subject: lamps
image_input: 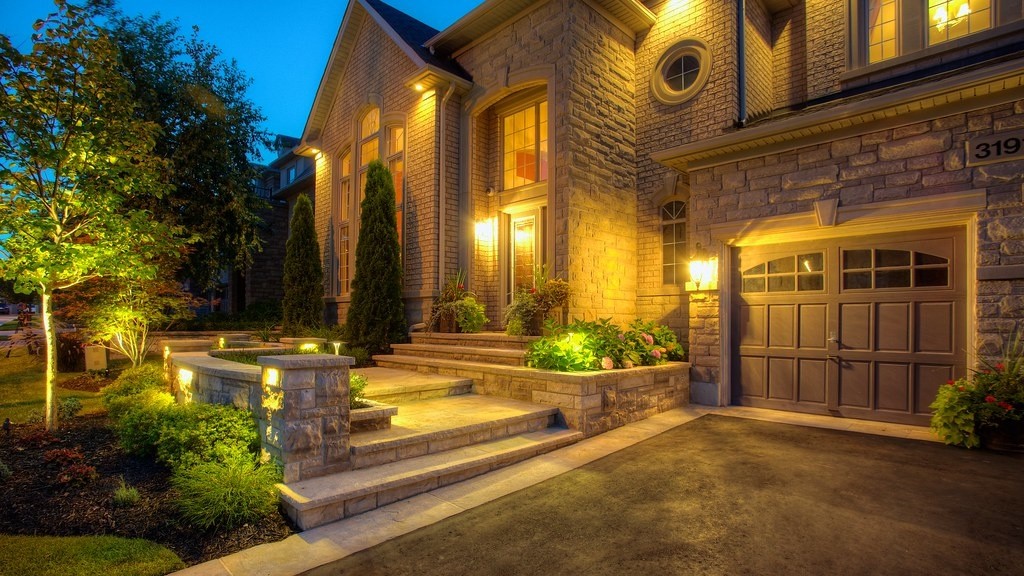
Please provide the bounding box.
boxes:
[934,0,971,32]
[689,242,708,292]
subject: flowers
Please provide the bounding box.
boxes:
[514,260,572,310]
[929,363,1024,449]
[525,316,687,370]
[431,267,479,307]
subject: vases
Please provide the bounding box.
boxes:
[530,310,564,335]
[440,307,457,333]
